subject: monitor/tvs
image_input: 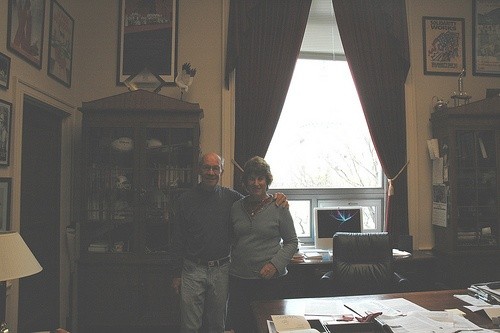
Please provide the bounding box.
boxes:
[313,208,364,249]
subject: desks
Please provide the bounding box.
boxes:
[246,285,500,333]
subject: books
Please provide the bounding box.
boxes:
[87,236,131,255]
[467,280,500,305]
[85,128,191,151]
[85,158,191,187]
[84,189,180,220]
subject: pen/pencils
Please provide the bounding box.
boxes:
[467,287,493,304]
[343,304,364,319]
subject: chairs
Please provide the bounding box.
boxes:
[319,229,408,291]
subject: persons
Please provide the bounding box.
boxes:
[229,155,300,333]
[167,150,286,332]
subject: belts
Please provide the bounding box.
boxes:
[185,255,231,267]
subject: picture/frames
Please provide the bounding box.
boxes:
[114,1,182,86]
[4,0,46,69]
[471,0,499,77]
[0,52,12,89]
[421,13,467,76]
[0,176,13,234]
[46,0,78,88]
[0,98,14,167]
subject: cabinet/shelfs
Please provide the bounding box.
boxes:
[429,111,500,284]
[74,90,201,332]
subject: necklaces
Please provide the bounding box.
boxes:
[245,195,267,217]
